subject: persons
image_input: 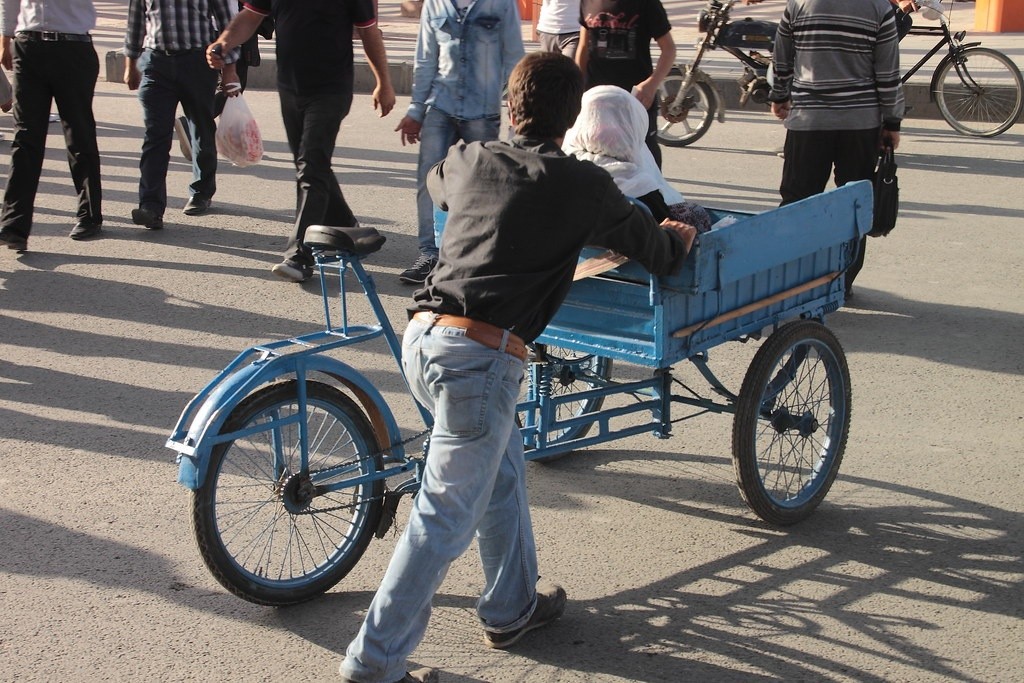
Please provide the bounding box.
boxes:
[0,0,105,252]
[766,0,918,302]
[563,85,723,243]
[339,50,695,683]
[574,0,677,176]
[205,0,397,284]
[124,0,264,230]
[395,0,528,289]
[536,0,582,61]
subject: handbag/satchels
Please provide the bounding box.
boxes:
[866,145,900,239]
[213,83,262,168]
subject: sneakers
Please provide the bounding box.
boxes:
[484,585,567,648]
[400,253,438,282]
[272,258,314,281]
[342,667,440,683]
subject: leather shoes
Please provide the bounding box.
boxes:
[0,228,28,250]
[183,197,212,213]
[69,220,101,239]
[132,208,163,229]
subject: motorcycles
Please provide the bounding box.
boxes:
[656,0,780,147]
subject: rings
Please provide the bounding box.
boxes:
[414,134,417,136]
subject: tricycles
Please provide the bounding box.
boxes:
[165,180,873,607]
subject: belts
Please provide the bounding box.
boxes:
[158,50,189,56]
[18,30,91,44]
[414,311,528,363]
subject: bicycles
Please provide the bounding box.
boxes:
[896,0,1024,137]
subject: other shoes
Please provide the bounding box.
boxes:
[844,288,853,299]
[175,115,193,161]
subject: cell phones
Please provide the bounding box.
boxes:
[213,44,224,76]
